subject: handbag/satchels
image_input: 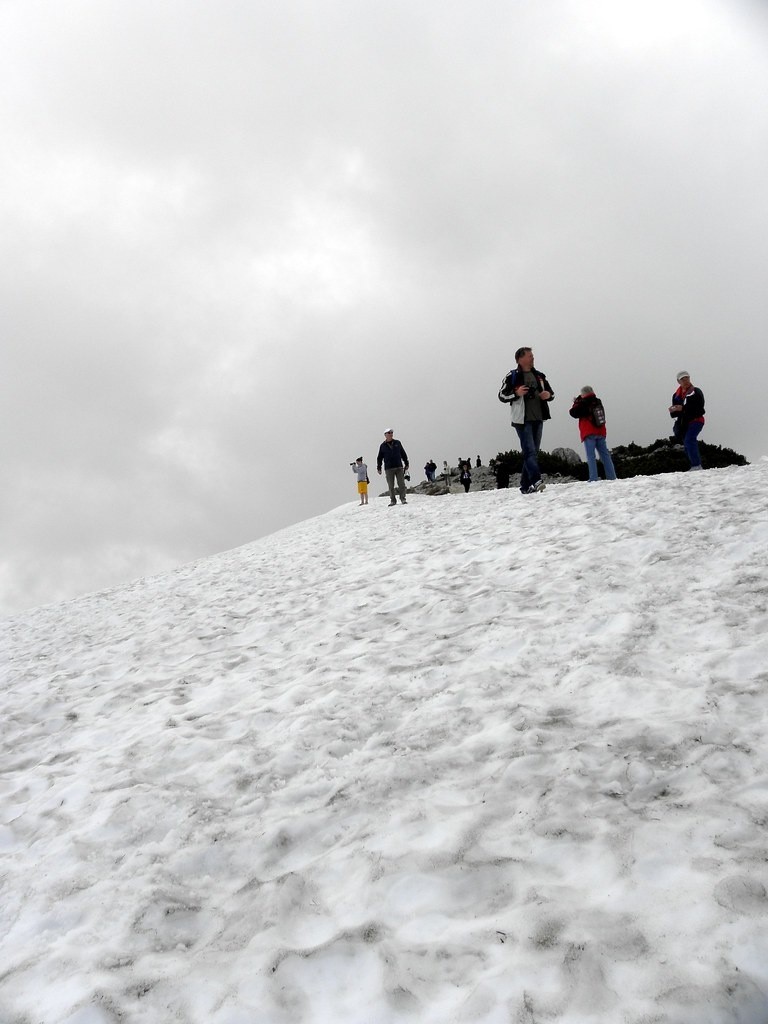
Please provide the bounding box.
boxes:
[366,477,370,484]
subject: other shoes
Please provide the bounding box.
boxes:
[388,501,396,506]
[360,502,364,505]
[401,499,407,504]
[673,444,684,451]
[365,502,368,504]
[690,465,703,471]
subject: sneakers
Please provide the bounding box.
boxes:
[520,484,536,494]
[534,480,546,492]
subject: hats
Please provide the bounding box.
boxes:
[677,370,689,381]
[384,429,393,434]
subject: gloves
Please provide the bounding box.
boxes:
[574,395,582,404]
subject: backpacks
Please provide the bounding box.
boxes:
[586,399,605,427]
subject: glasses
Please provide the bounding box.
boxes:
[387,432,393,434]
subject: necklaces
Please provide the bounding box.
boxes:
[388,442,393,449]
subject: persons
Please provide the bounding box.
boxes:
[442,461,452,486]
[493,457,509,489]
[424,460,437,481]
[352,457,368,506]
[460,464,472,493]
[377,428,409,506]
[569,386,616,482]
[498,347,555,494]
[476,455,481,467]
[458,457,471,470]
[669,371,706,471]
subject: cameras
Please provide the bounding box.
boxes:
[405,475,410,481]
[350,462,356,465]
[672,406,676,411]
[524,385,535,400]
